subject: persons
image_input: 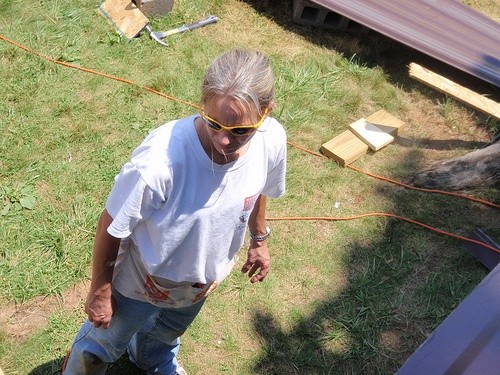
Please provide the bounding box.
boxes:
[59,48,289,375]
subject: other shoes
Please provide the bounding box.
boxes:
[174,361,189,375]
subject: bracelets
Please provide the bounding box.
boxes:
[250,226,271,241]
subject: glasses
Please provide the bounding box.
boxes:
[199,91,268,136]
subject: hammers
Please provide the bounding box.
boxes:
[145,15,219,47]
